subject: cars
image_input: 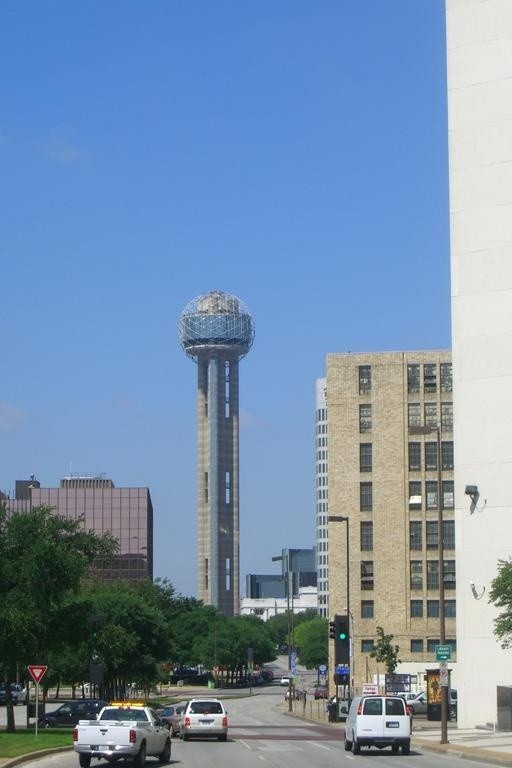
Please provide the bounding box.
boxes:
[0,683,27,706]
[158,703,186,736]
[78,681,98,691]
[172,666,200,675]
[314,684,328,699]
[284,687,306,701]
[36,697,112,727]
[395,690,416,701]
[280,675,289,685]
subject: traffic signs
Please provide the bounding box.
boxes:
[435,645,451,660]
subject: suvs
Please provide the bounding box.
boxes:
[405,688,457,717]
[177,696,230,741]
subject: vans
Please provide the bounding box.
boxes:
[341,690,413,756]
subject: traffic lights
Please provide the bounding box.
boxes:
[339,619,347,641]
[329,619,336,639]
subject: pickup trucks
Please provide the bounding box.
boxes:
[72,705,172,768]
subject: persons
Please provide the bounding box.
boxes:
[431,679,439,702]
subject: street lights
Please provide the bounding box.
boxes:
[324,514,354,702]
[408,422,452,744]
[271,554,292,671]
